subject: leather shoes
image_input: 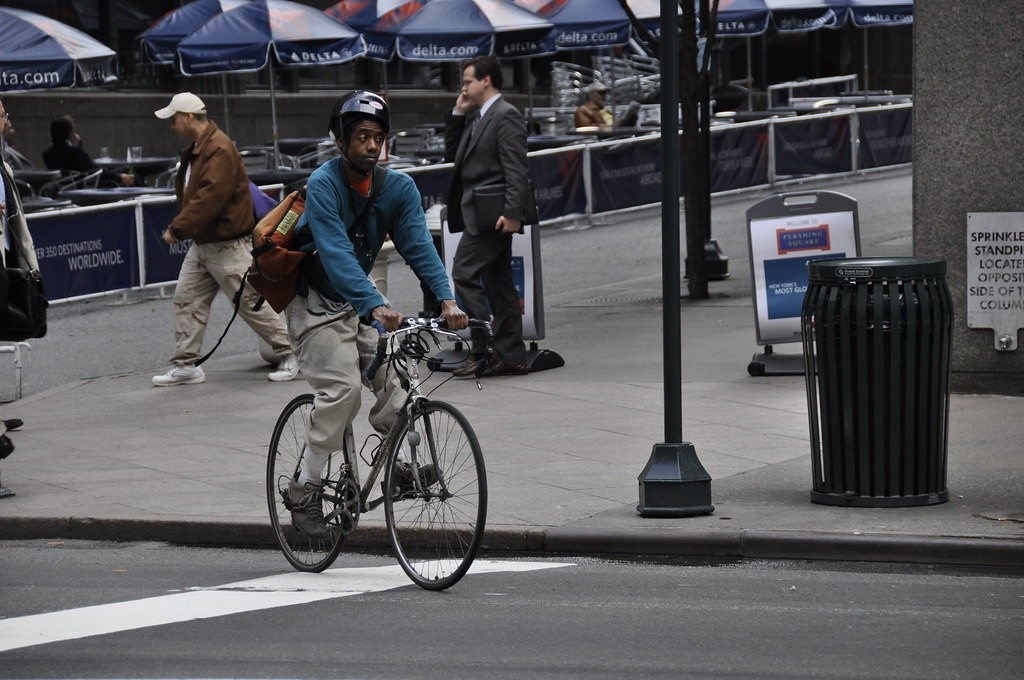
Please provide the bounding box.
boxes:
[481,360,529,376]
[453,357,488,375]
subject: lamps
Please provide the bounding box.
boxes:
[265,137,332,145]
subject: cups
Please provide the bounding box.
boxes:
[100,147,109,161]
[132,147,142,161]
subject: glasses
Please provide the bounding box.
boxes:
[595,90,608,95]
[0,112,9,121]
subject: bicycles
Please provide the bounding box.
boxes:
[264,320,498,593]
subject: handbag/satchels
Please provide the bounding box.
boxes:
[0,211,49,341]
[246,180,318,314]
[248,180,279,222]
[473,178,540,235]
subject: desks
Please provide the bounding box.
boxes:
[716,109,798,117]
[93,157,177,165]
[416,122,446,128]
[246,167,314,175]
[566,125,663,133]
[20,198,73,208]
[74,187,174,194]
[526,133,598,145]
[379,157,423,167]
[12,167,61,176]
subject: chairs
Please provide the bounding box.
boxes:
[12,80,911,214]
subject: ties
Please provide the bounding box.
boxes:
[471,114,480,137]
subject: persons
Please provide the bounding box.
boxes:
[575,82,616,126]
[0,101,40,459]
[42,114,135,188]
[440,56,532,376]
[152,90,300,387]
[289,89,470,538]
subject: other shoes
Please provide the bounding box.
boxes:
[0,434,15,460]
[5,419,25,431]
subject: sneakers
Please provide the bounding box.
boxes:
[384,462,442,502]
[151,363,206,387]
[267,359,299,381]
[279,471,330,539]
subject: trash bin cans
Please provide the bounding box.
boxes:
[800,255,955,508]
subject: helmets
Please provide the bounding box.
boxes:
[329,89,391,146]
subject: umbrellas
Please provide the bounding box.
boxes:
[0,5,118,92]
[138,0,912,171]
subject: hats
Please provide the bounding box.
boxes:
[585,82,612,94]
[153,91,207,120]
